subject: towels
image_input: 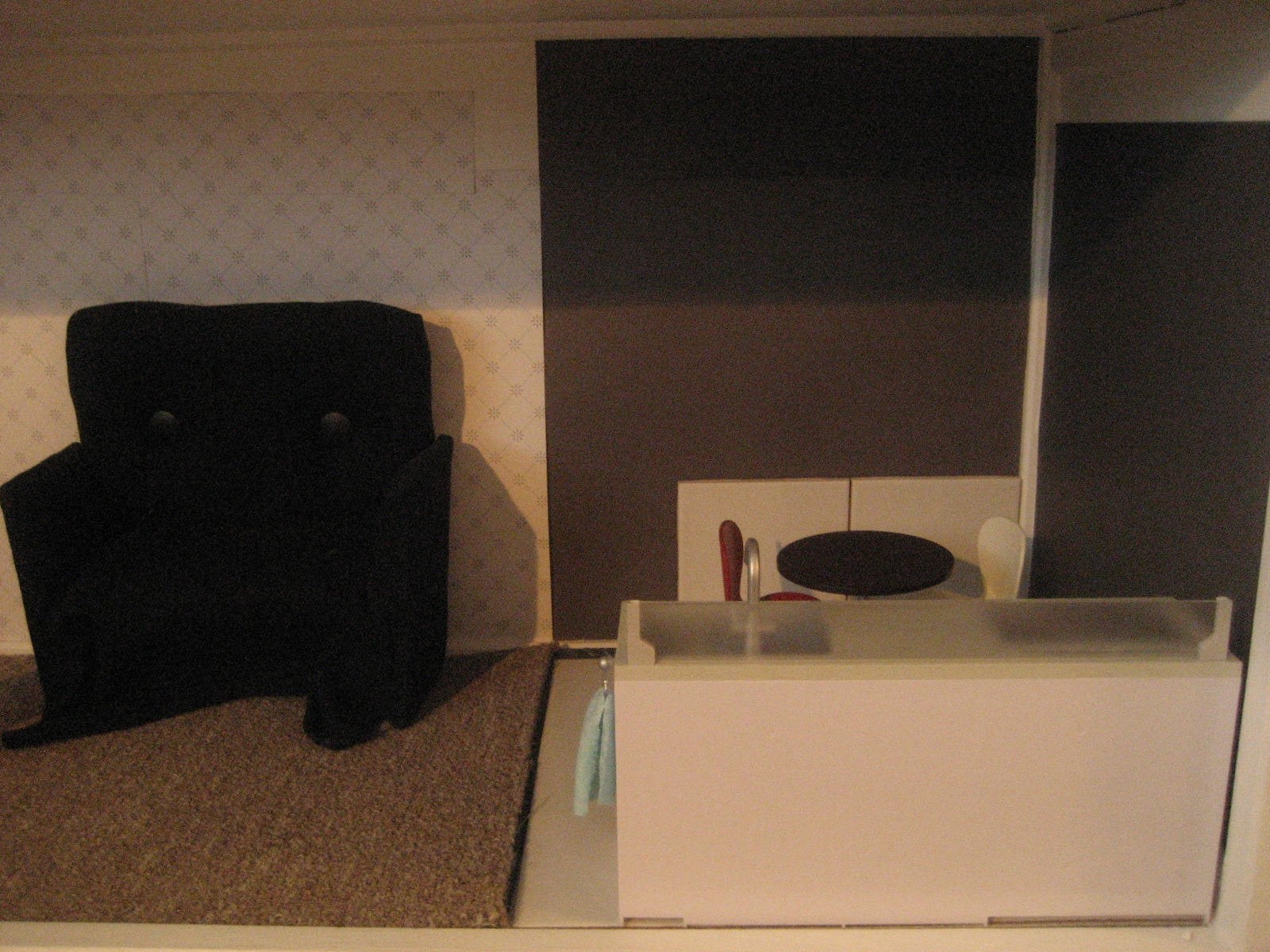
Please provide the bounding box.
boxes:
[571,687,615,819]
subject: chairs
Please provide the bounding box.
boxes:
[718,520,819,602]
[977,518,1027,600]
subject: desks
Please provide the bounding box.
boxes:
[774,530,954,603]
[615,596,1244,927]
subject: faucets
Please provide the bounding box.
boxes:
[742,536,760,604]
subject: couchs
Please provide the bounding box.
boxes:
[0,296,454,749]
